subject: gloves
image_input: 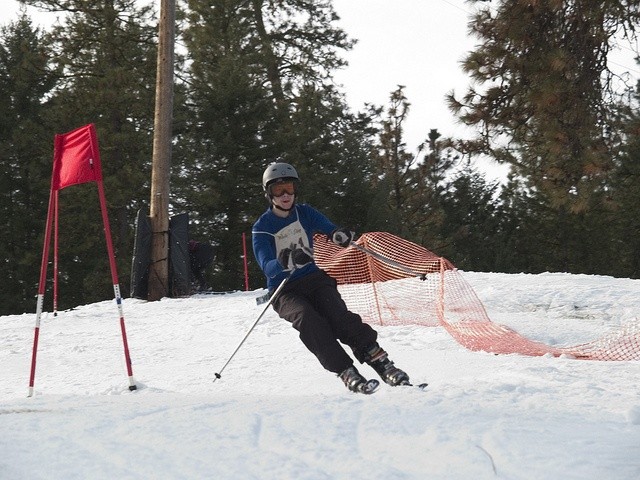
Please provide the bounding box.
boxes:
[330,228,351,249]
[278,247,314,271]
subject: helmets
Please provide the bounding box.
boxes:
[262,163,298,191]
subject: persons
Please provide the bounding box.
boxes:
[252,162,410,390]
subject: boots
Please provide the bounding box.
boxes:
[338,366,362,392]
[363,341,405,385]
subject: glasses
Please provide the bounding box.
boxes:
[268,180,297,197]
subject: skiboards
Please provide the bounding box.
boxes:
[360,379,428,394]
